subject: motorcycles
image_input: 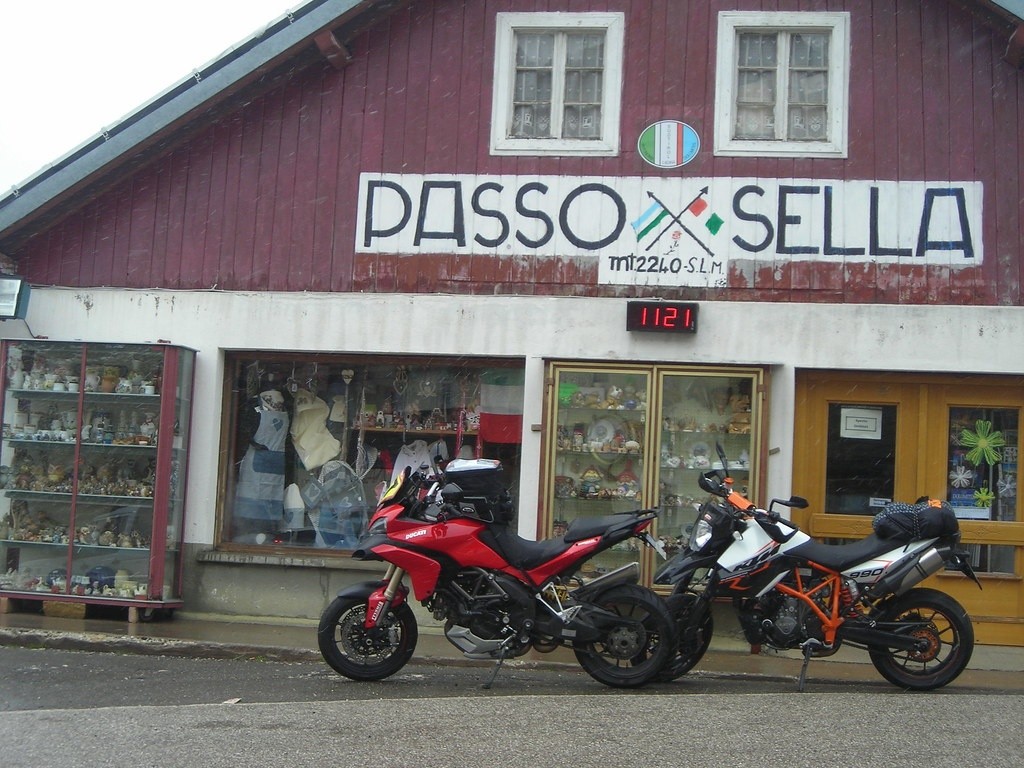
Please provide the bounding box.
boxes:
[629,440,984,693]
[316,439,678,691]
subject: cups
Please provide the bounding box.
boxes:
[728,461,740,466]
[60,431,69,440]
[53,383,64,391]
[145,386,154,394]
[69,383,78,392]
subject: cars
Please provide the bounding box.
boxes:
[16,505,356,585]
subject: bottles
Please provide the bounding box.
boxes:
[115,569,128,589]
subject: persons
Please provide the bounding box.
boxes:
[233,389,289,520]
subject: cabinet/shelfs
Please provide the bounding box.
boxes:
[0,339,201,608]
[557,373,753,603]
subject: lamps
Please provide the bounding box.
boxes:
[0,274,31,320]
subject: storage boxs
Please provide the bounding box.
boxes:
[728,412,751,434]
